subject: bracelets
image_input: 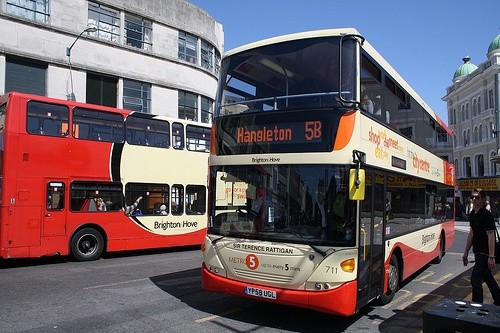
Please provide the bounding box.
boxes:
[488,256,496,259]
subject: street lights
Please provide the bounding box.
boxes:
[67,27,98,57]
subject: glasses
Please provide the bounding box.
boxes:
[470,196,483,199]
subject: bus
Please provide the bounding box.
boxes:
[202,27,456,318]
[0,92,213,263]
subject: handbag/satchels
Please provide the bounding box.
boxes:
[386,212,394,221]
[495,242,500,264]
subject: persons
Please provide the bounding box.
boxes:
[385,198,394,223]
[310,187,348,241]
[359,88,390,125]
[465,196,500,218]
[434,200,453,219]
[463,189,500,307]
[247,184,280,229]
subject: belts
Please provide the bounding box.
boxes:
[475,252,489,256]
